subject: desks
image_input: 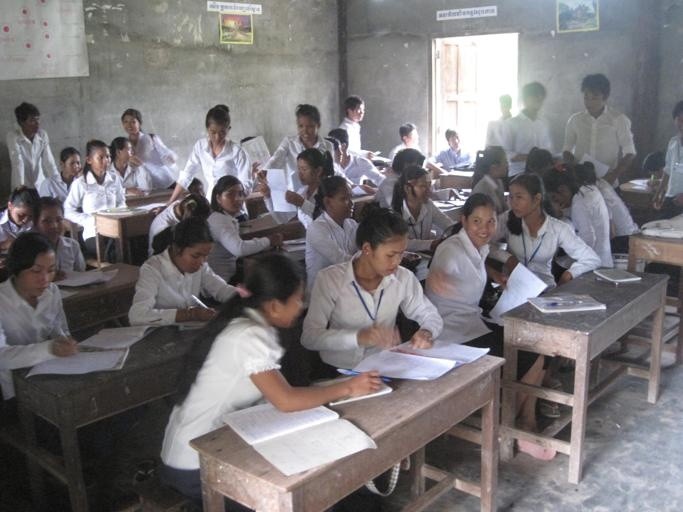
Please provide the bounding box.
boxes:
[239,214,306,241]
[60,189,189,241]
[620,212,682,365]
[437,170,474,189]
[57,262,140,334]
[619,179,651,209]
[90,203,166,264]
[352,194,373,223]
[432,192,509,221]
[410,250,432,283]
[245,191,268,220]
[188,355,507,512]
[239,245,304,275]
[496,266,669,485]
[9,322,203,512]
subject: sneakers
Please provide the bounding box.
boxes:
[515,440,556,460]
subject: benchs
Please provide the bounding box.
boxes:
[138,485,191,512]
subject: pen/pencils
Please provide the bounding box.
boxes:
[336,368,393,383]
[59,328,69,339]
[191,293,210,310]
[595,277,619,286]
[546,302,576,306]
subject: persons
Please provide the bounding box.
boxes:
[485,94,513,148]
[565,161,642,256]
[560,73,638,195]
[388,122,423,163]
[297,200,445,384]
[0,230,144,512]
[152,104,253,221]
[257,147,335,230]
[371,148,425,214]
[205,174,284,287]
[0,183,39,255]
[324,136,378,196]
[304,176,420,305]
[643,151,667,193]
[338,95,378,161]
[489,172,604,419]
[388,163,454,253]
[108,136,150,196]
[647,100,683,297]
[430,128,473,173]
[39,146,82,204]
[6,101,61,199]
[499,81,553,179]
[120,108,179,189]
[423,192,559,462]
[148,192,211,258]
[250,103,357,195]
[472,145,511,213]
[128,216,239,328]
[523,145,554,194]
[157,252,382,512]
[31,195,124,330]
[544,164,616,283]
[62,139,128,262]
[328,128,387,187]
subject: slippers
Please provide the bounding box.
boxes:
[540,402,562,417]
[131,457,155,484]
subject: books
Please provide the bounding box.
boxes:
[279,240,307,252]
[76,346,130,373]
[222,400,378,479]
[77,325,157,348]
[593,269,641,288]
[312,376,393,405]
[527,294,607,315]
[98,208,147,217]
[434,200,465,211]
